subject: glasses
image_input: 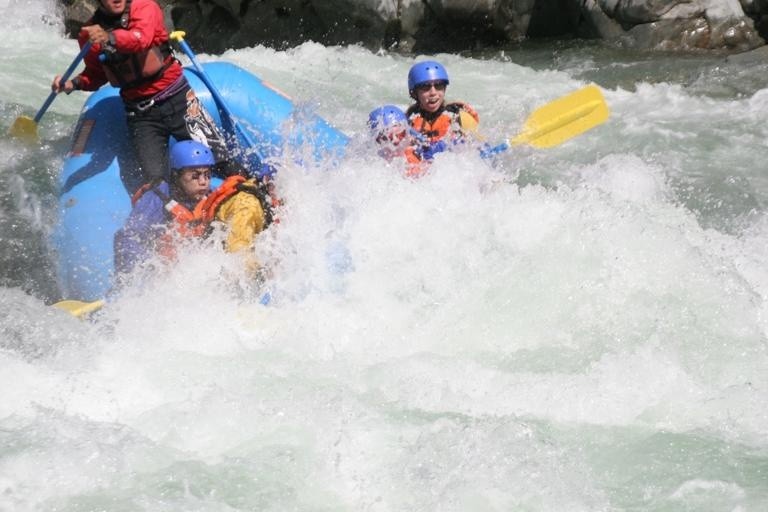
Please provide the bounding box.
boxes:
[177,172,210,181]
[419,83,446,92]
[381,130,407,142]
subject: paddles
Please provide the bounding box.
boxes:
[8,39,93,143]
[479,83,610,160]
[50,300,106,318]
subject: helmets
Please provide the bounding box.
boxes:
[168,141,216,184]
[367,105,408,132]
[408,61,449,98]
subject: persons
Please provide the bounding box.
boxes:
[200,162,285,280]
[50,0,251,186]
[399,59,480,140]
[111,133,215,278]
[366,106,437,181]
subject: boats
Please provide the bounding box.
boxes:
[52,62,355,303]
